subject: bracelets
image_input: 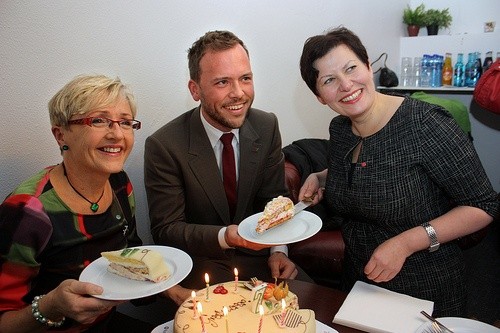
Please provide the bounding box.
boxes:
[31,295,65,327]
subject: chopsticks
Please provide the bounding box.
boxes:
[420,311,454,333]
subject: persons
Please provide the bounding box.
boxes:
[0,75,200,333]
[143,31,314,290]
[298,27,500,319]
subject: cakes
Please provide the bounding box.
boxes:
[257,196,294,231]
[100,248,172,283]
[172,277,317,333]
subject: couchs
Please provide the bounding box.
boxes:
[285,159,345,256]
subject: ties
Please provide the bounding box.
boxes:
[220,134,238,220]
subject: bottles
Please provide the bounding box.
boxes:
[453,53,465,87]
[483,51,500,73]
[442,52,452,86]
[430,53,444,87]
[419,54,432,87]
[465,52,482,88]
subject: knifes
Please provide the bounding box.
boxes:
[293,191,318,216]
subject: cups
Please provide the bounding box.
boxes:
[400,57,412,87]
[413,57,423,86]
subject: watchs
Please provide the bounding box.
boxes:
[421,222,440,252]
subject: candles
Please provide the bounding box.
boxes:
[223,306,229,333]
[251,282,267,313]
[197,302,206,333]
[280,299,286,326]
[205,273,209,299]
[234,268,238,290]
[191,291,196,319]
[257,305,264,333]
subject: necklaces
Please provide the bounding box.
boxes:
[62,161,104,212]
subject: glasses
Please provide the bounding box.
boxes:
[66,116,141,130]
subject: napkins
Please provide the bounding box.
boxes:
[331,280,434,333]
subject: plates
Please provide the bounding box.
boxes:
[79,245,193,300]
[238,210,323,245]
[415,317,500,333]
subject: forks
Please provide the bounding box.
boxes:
[424,322,443,333]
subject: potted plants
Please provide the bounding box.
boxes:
[402,2,452,37]
